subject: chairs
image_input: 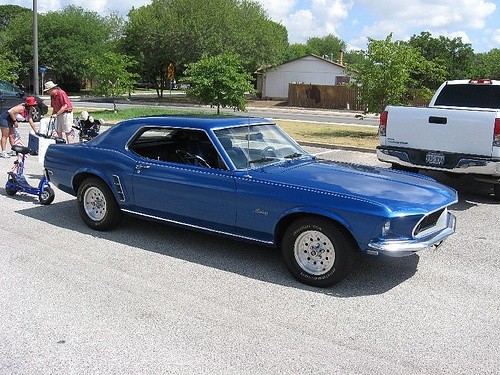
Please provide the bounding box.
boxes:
[217,138,247,170]
[247,132,275,165]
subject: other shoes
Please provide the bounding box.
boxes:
[7,149,17,156]
[0,151,11,158]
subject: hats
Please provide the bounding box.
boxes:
[25,96,39,106]
[42,80,57,92]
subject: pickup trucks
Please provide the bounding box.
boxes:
[374,78,500,200]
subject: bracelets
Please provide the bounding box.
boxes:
[13,120,16,122]
[57,112,60,116]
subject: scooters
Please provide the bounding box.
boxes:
[5,135,67,206]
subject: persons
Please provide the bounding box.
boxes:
[43,81,73,144]
[0,97,38,158]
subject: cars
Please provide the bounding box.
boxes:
[43,113,460,290]
[0,78,49,123]
